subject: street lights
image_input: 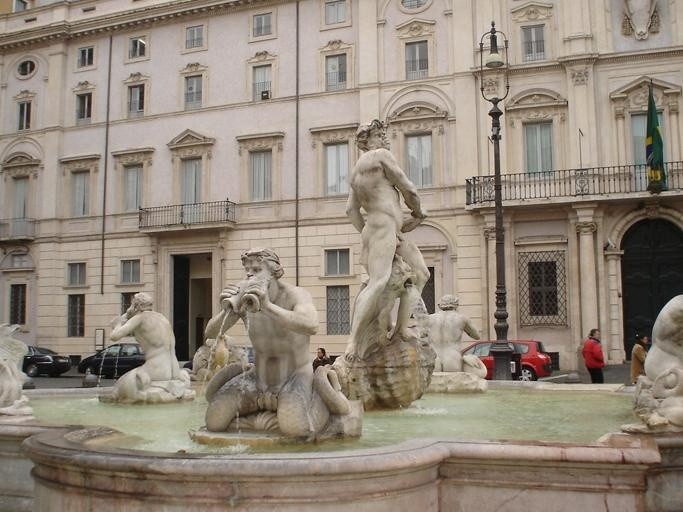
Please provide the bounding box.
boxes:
[480,22,513,380]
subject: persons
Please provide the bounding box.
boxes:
[110,291,190,398]
[203,247,351,436]
[428,295,488,379]
[312,348,332,372]
[343,118,432,363]
[631,331,649,385]
[581,328,606,382]
[632,295,683,407]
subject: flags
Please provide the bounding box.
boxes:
[645,84,667,195]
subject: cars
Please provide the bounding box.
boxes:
[22,346,71,378]
[461,340,552,381]
[77,343,146,378]
